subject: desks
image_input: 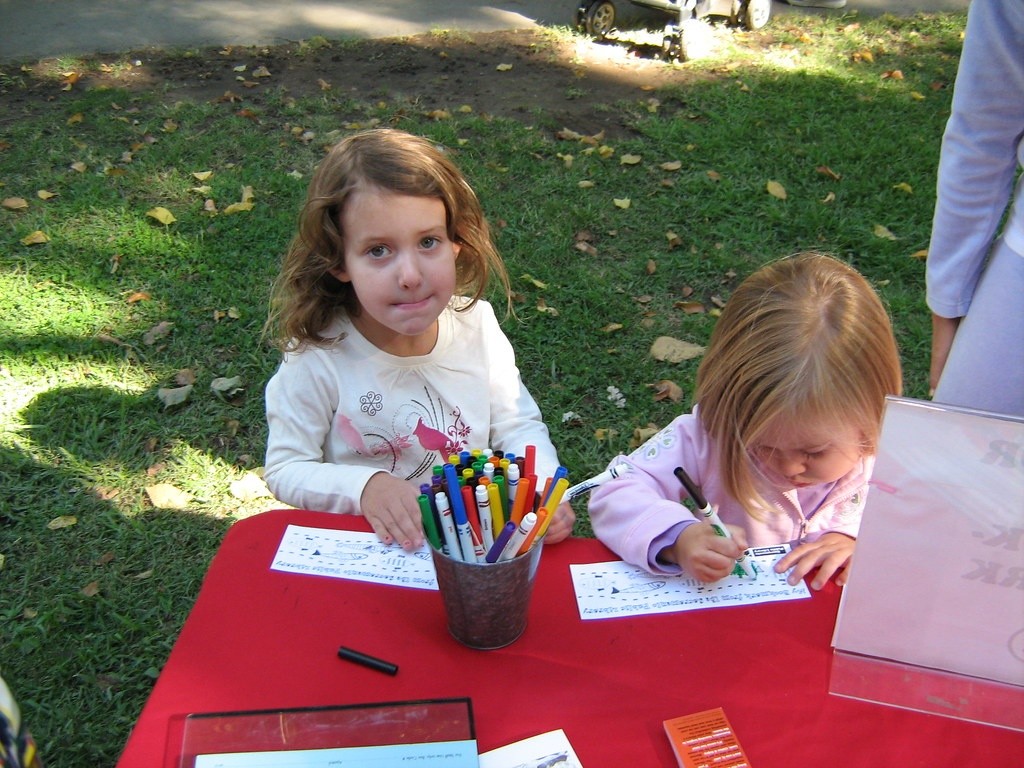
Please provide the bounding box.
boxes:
[116,511,1024,768]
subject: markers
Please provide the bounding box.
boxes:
[673,465,757,583]
[416,444,628,564]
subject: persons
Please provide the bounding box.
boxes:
[587,250,903,591]
[260,123,576,551]
[925,0,1024,418]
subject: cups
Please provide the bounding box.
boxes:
[420,512,547,649]
